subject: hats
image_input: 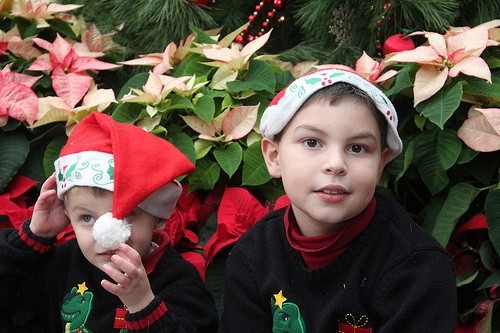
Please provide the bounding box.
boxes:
[54,112,196,251]
[259,65,403,161]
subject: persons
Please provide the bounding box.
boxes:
[0,113,219,333]
[218,68,458,333]
